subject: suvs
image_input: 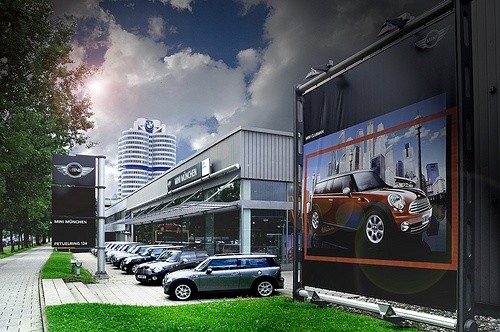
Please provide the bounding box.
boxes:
[93,241,209,283]
[312,169,434,246]
[162,253,284,299]
